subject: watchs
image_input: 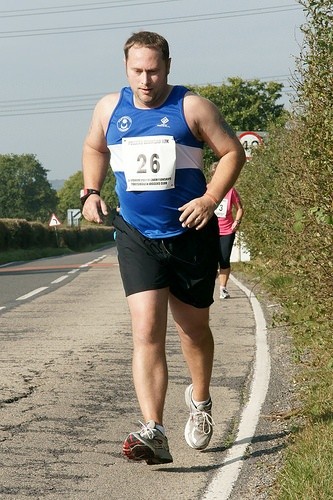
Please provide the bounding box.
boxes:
[80,188,101,205]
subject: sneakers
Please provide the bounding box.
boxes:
[185,383,215,450]
[122,420,173,466]
[219,290,230,299]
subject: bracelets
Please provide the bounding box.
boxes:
[204,193,220,207]
[234,219,242,224]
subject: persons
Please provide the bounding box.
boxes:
[79,30,247,465]
[205,161,244,299]
